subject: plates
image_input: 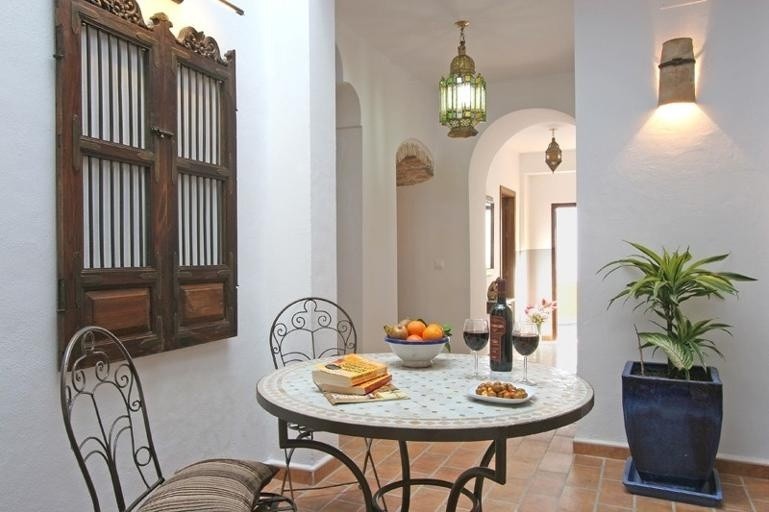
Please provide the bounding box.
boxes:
[468,382,537,405]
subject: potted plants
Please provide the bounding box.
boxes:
[596,238,759,508]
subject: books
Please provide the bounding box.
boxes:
[312,354,411,405]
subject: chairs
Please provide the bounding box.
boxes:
[269,296,388,512]
[60,325,297,512]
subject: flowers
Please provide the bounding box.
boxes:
[524,297,558,335]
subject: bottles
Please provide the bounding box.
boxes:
[490,278,514,372]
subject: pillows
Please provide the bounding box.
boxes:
[135,458,280,512]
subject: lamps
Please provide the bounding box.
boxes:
[657,37,697,109]
[438,21,487,138]
[545,128,562,173]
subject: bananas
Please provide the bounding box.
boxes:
[384,325,392,336]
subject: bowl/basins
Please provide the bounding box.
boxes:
[385,335,448,367]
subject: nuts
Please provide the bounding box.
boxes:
[476,381,528,398]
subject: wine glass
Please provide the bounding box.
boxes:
[463,318,490,380]
[512,322,539,386]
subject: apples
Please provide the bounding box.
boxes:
[399,320,409,326]
[392,324,408,340]
